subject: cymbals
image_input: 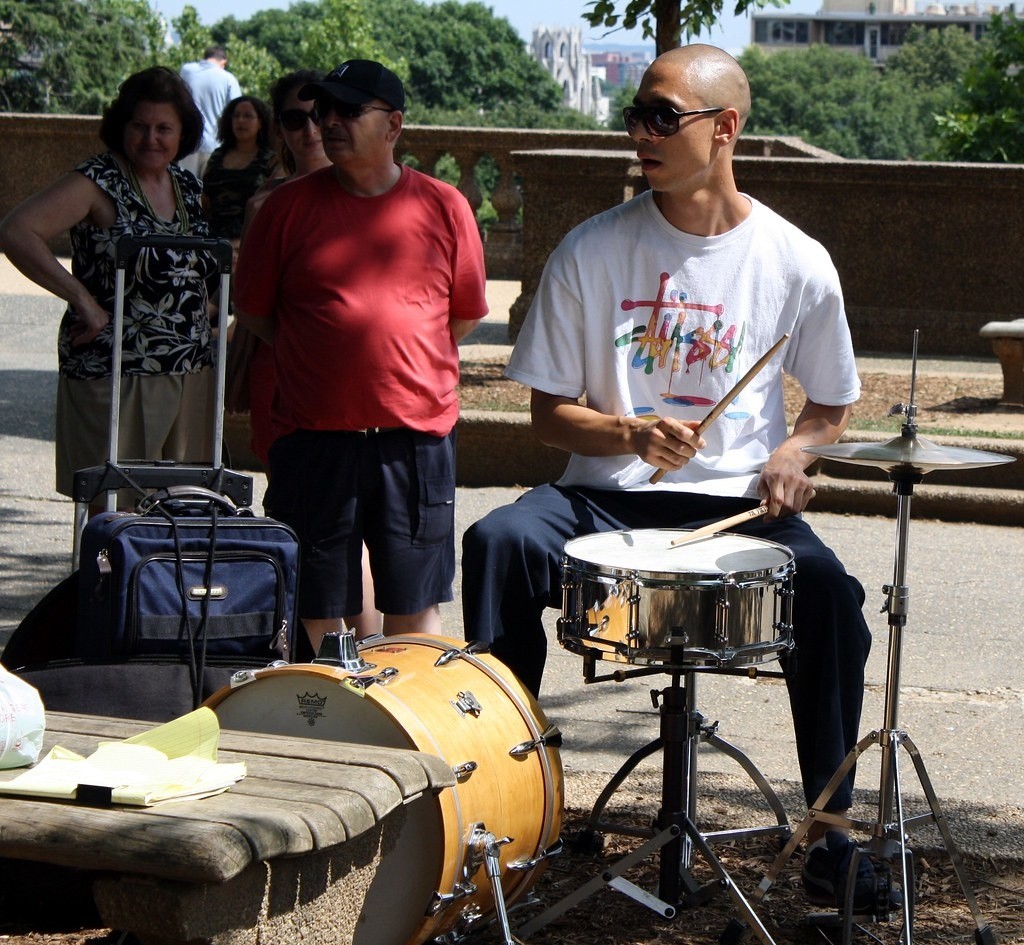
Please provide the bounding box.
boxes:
[800,430,1019,483]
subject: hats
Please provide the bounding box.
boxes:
[297,59,405,113]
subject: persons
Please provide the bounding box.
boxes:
[230,59,488,654]
[462,43,910,916]
[1,65,363,656]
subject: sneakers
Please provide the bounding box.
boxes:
[801,829,904,915]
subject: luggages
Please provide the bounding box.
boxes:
[0,233,301,723]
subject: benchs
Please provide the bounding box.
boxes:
[0,710,457,945]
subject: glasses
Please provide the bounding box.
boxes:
[314,99,396,118]
[622,105,726,136]
[278,108,321,132]
[233,110,257,119]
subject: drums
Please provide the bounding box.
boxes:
[193,637,565,945]
[556,525,794,666]
